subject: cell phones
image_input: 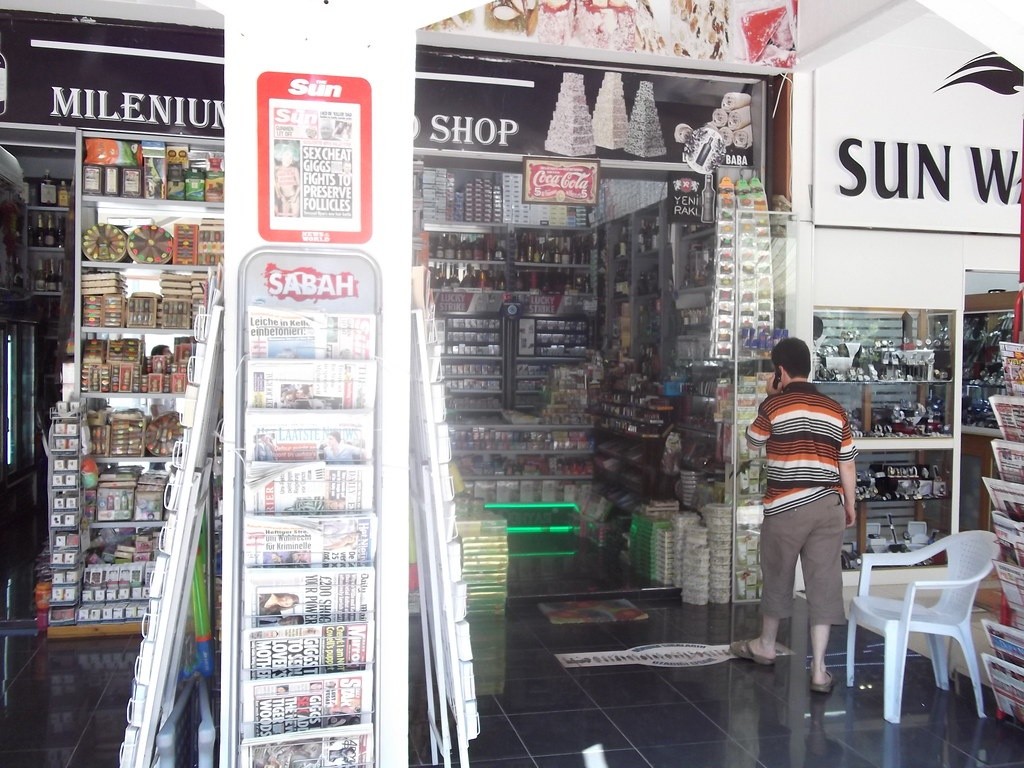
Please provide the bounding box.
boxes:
[773,370,782,390]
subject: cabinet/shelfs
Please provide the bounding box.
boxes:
[598,194,763,476]
[446,416,595,519]
[73,128,226,563]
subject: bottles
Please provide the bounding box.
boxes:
[45,258,56,292]
[97,493,107,510]
[114,492,120,510]
[121,491,128,509]
[28,214,35,246]
[43,171,51,185]
[35,213,44,247]
[434,269,590,295]
[129,491,133,511]
[44,214,56,247]
[205,158,225,202]
[430,234,587,264]
[35,258,45,292]
[58,181,68,206]
[107,492,114,510]
[56,215,65,248]
[615,217,657,299]
[57,259,64,291]
[696,137,714,221]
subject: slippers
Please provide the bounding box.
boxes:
[731,639,776,664]
[810,670,834,692]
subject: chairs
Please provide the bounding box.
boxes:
[846,529,1001,724]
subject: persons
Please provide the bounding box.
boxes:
[255,386,367,768]
[728,337,861,694]
